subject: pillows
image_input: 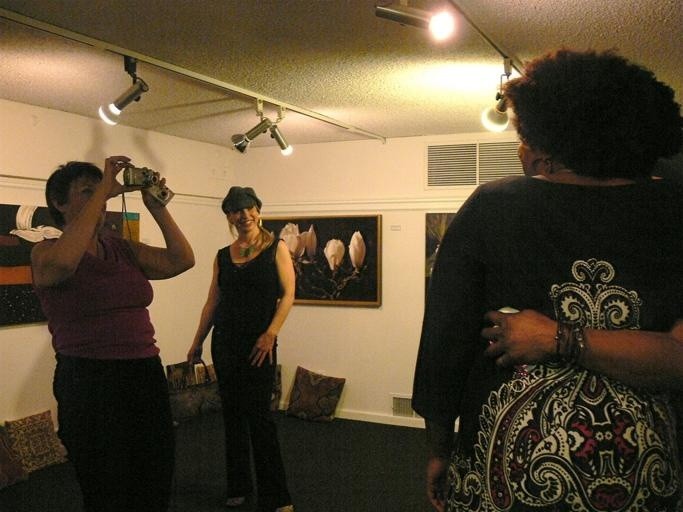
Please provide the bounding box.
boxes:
[197,365,219,382]
[0,426,32,487]
[269,365,282,412]
[286,364,345,424]
[5,411,66,471]
[167,360,196,388]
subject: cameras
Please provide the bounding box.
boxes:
[124,166,161,186]
[146,183,175,207]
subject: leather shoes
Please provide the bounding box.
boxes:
[276,504,294,512]
[226,497,245,506]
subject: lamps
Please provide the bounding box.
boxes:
[231,100,272,154]
[269,106,292,157]
[376,2,454,41]
[482,59,510,131]
[98,57,148,125]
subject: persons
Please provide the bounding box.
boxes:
[187,185,297,512]
[480,308,683,394]
[410,49,683,512]
[29,160,197,512]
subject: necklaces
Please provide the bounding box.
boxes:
[237,240,257,256]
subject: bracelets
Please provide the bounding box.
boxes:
[554,319,587,365]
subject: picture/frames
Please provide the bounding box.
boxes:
[257,214,383,308]
[0,204,141,329]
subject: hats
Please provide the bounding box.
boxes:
[222,186,262,213]
